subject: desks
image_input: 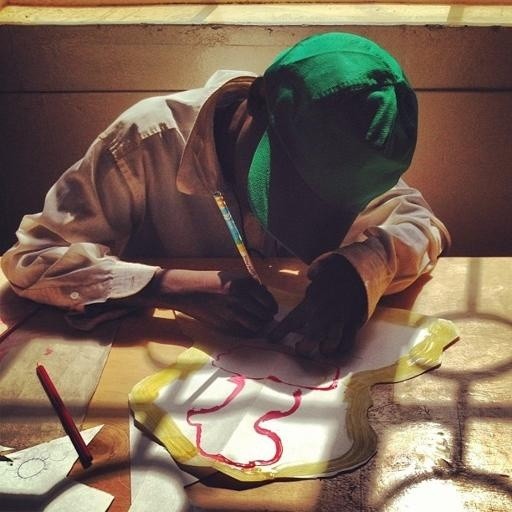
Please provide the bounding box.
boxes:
[0,255,512,511]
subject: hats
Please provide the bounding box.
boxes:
[245,31,420,267]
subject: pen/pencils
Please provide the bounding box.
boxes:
[36,361,92,464]
[212,190,262,286]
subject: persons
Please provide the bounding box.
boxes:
[0,32,452,359]
